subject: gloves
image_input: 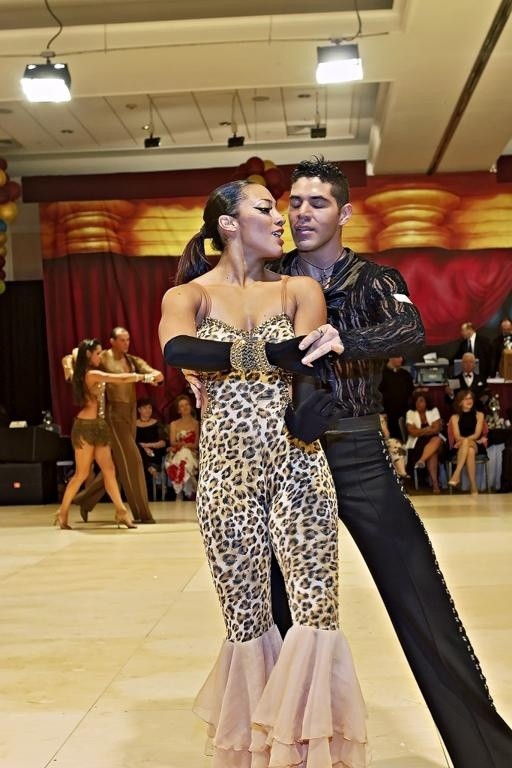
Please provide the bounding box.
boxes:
[281,372,343,444]
[161,331,331,378]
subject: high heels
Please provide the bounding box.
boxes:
[114,509,138,531]
[53,509,74,531]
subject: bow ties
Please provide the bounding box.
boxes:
[463,373,473,379]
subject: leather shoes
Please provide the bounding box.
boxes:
[132,519,156,524]
[72,494,91,523]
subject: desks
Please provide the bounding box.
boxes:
[481,411,512,492]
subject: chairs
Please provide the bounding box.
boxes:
[397,413,451,493]
[161,450,172,500]
[141,436,159,497]
[449,442,491,493]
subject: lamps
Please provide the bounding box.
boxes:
[314,37,364,90]
[16,0,76,106]
[225,93,246,149]
[143,96,161,150]
[308,89,327,138]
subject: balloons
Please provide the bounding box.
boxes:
[0,159,21,294]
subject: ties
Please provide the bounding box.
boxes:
[467,339,473,353]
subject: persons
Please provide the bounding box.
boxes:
[158,179,370,767]
[262,155,512,768]
